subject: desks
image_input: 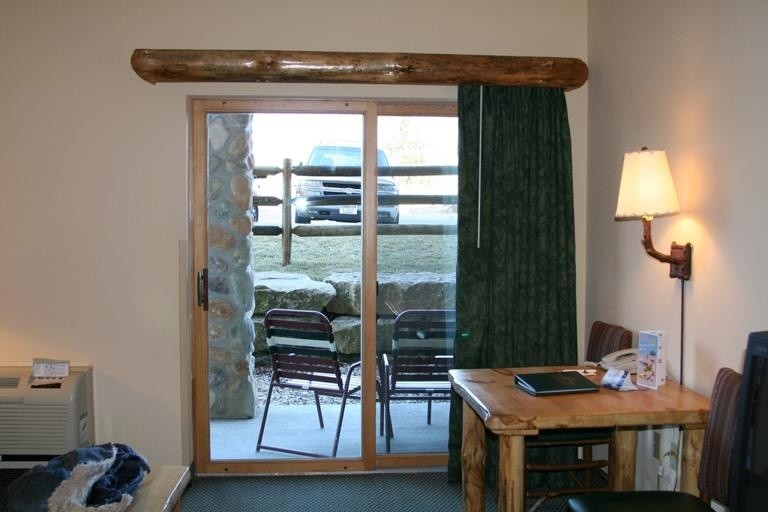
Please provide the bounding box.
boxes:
[449,366,714,512]
[124,465,192,512]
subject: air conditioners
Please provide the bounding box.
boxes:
[0,365,94,470]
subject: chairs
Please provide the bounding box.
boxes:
[526,320,632,499]
[256,309,385,457]
[380,309,459,453]
[568,368,743,512]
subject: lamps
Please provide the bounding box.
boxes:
[614,147,690,279]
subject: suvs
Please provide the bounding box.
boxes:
[289,143,402,226]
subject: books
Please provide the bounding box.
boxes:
[513,371,599,396]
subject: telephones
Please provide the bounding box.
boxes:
[600,348,639,374]
